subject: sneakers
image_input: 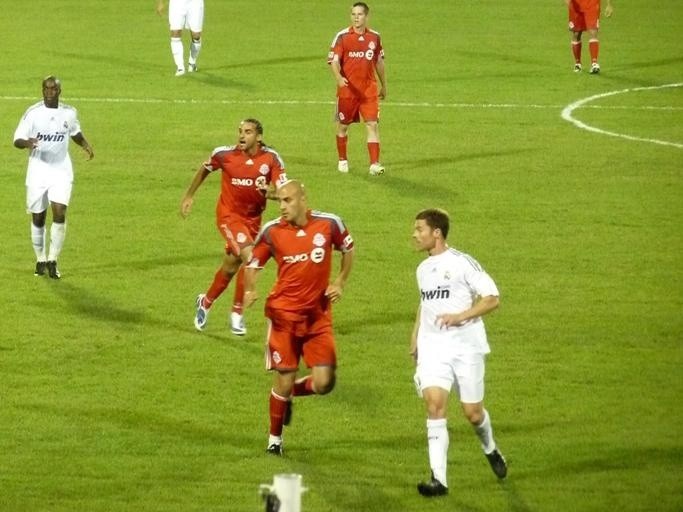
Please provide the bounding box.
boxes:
[286,397,292,426]
[194,293,210,332]
[266,439,284,454]
[370,163,385,176]
[34,260,61,280]
[338,161,349,172]
[416,478,448,496]
[231,314,246,334]
[485,444,507,479]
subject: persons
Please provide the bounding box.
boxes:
[407,208,507,496]
[242,180,355,458]
[11,75,95,278]
[156,0,207,77]
[564,0,613,75]
[181,119,288,335]
[326,1,387,176]
